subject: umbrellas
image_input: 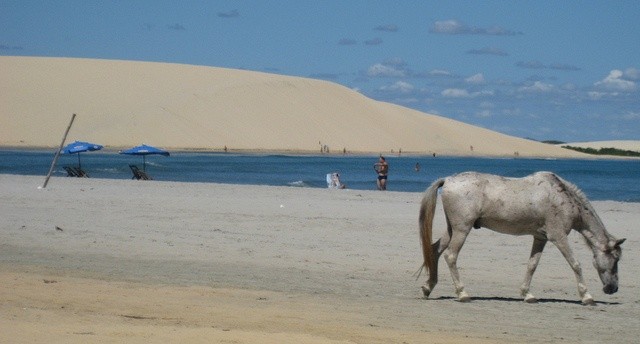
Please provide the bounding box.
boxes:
[119,144,169,174]
[55,140,103,174]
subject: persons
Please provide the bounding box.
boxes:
[373,157,388,191]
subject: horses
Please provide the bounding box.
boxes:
[412,170,628,306]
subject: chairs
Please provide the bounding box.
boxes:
[73,166,88,177]
[128,165,151,180]
[63,167,78,177]
